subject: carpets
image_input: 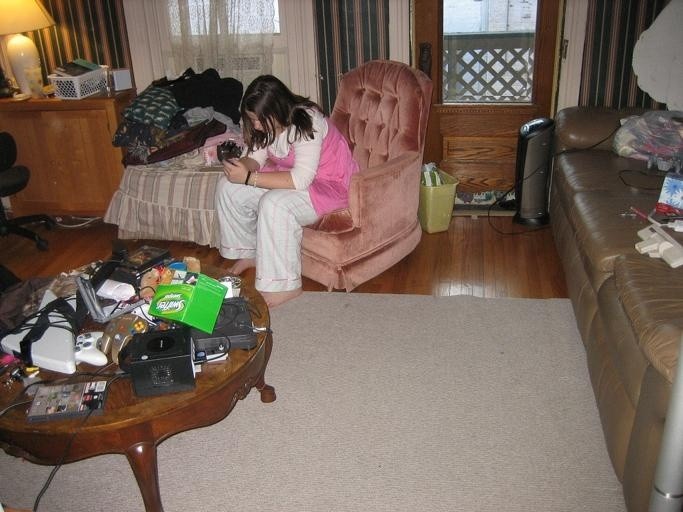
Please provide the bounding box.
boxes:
[1,290,626,511]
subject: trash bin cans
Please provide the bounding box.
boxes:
[417,167,459,234]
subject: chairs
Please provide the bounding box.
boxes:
[1,132,58,251]
[299,61,434,294]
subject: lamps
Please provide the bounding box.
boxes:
[0,0,55,102]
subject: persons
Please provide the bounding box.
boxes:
[214,75,360,309]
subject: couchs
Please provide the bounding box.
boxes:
[544,104,683,512]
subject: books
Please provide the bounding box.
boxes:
[148,268,228,335]
[26,381,107,421]
[53,58,102,78]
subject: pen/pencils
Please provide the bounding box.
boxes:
[630,206,662,227]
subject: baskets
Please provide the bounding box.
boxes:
[46,63,111,102]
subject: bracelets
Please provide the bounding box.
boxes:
[245,170,258,188]
